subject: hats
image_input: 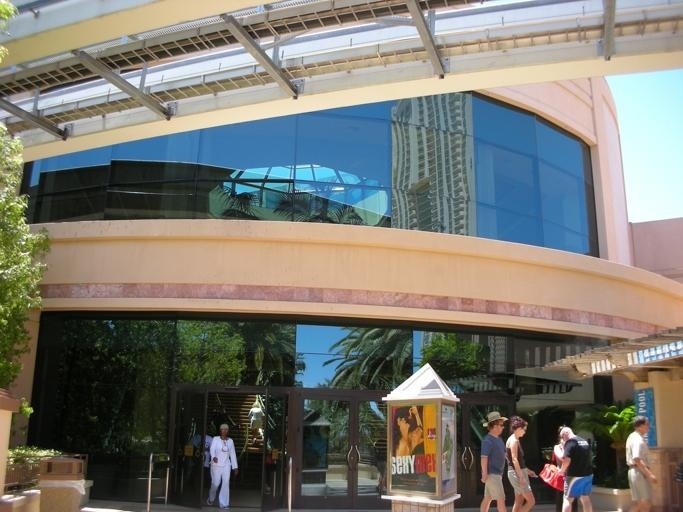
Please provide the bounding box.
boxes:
[483,411,508,427]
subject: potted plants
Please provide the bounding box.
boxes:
[570,398,637,477]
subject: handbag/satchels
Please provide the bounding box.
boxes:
[539,464,564,491]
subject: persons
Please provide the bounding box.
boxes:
[247,400,265,436]
[206,424,239,509]
[406,425,424,455]
[555,427,595,512]
[479,411,508,512]
[504,416,539,511]
[191,426,211,492]
[625,415,657,512]
[550,425,578,512]
[394,405,424,456]
